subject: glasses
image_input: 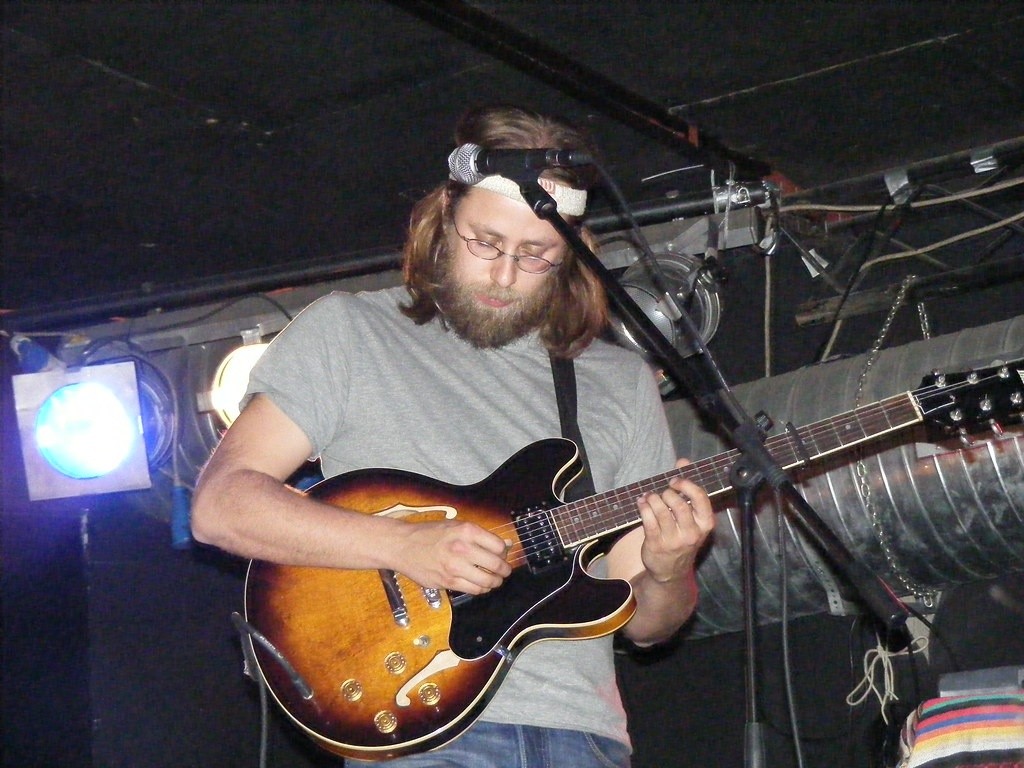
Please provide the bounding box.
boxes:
[450,213,563,274]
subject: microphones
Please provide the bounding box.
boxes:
[447,143,592,186]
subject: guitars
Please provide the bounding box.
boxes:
[234,353,1023,759]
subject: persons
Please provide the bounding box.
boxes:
[189,107,717,768]
